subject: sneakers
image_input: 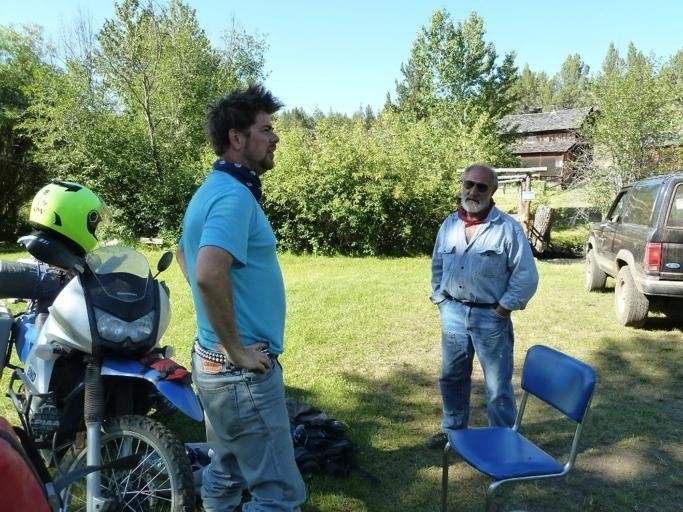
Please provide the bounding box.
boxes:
[427,433,448,449]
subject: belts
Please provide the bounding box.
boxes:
[443,290,497,309]
[193,340,271,363]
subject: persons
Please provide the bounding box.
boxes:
[176,84,307,512]
[426,165,539,450]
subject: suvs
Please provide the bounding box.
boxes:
[584,174,682,328]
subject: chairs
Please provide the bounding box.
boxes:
[435,343,598,511]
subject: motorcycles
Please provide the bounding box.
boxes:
[0,229,203,511]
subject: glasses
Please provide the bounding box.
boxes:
[464,181,488,192]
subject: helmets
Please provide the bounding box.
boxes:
[29,180,112,255]
